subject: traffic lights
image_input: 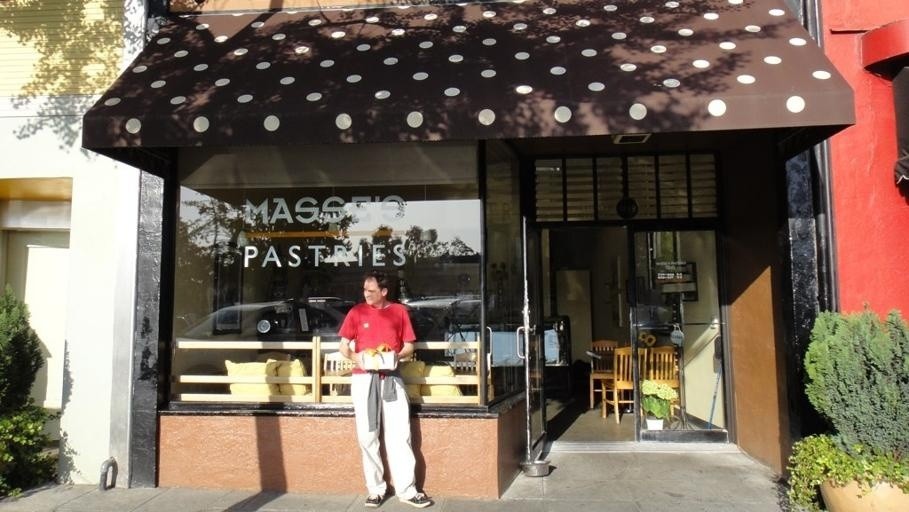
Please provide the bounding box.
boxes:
[224,353,309,401]
[396,355,465,401]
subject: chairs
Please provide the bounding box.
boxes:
[365,494,382,507]
[400,493,431,508]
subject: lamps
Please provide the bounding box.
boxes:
[585,339,680,425]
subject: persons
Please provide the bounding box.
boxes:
[338,270,431,508]
